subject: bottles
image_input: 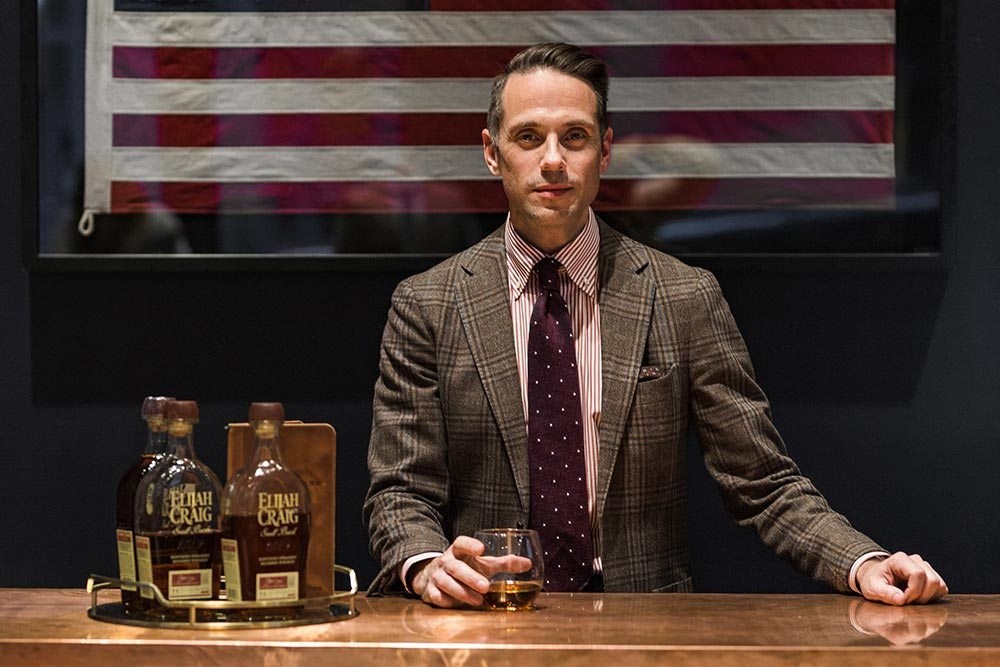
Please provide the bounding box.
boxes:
[135,401,225,621]
[115,395,177,618]
[219,402,310,621]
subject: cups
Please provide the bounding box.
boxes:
[473,528,545,612]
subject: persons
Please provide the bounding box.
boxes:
[362,39,952,613]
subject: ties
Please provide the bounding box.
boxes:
[528,257,594,589]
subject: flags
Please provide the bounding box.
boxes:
[77,0,900,235]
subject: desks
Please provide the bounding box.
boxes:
[0,588,1000,667]
